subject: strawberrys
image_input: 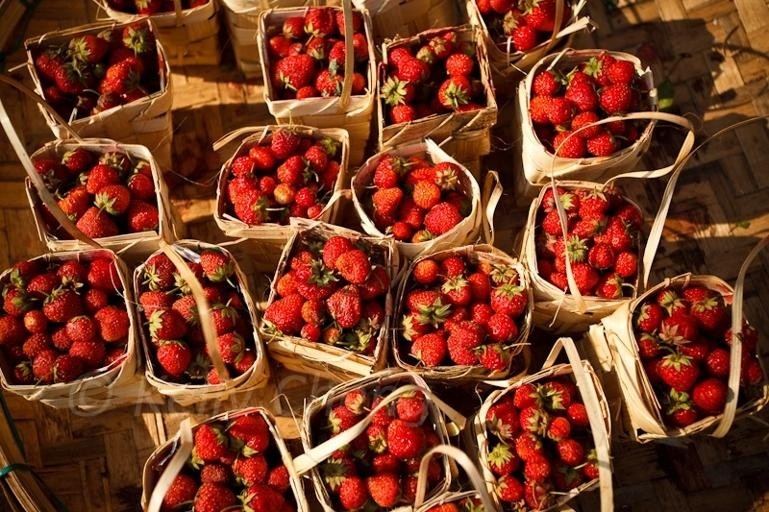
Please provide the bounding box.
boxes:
[0,2,763,512]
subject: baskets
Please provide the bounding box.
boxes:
[141,406,308,512]
[0,248,166,418]
[92,3,225,73]
[24,12,173,178]
[459,359,615,512]
[299,368,458,512]
[24,137,188,267]
[214,0,694,386]
[214,1,697,386]
[133,239,272,406]
[572,273,769,451]
